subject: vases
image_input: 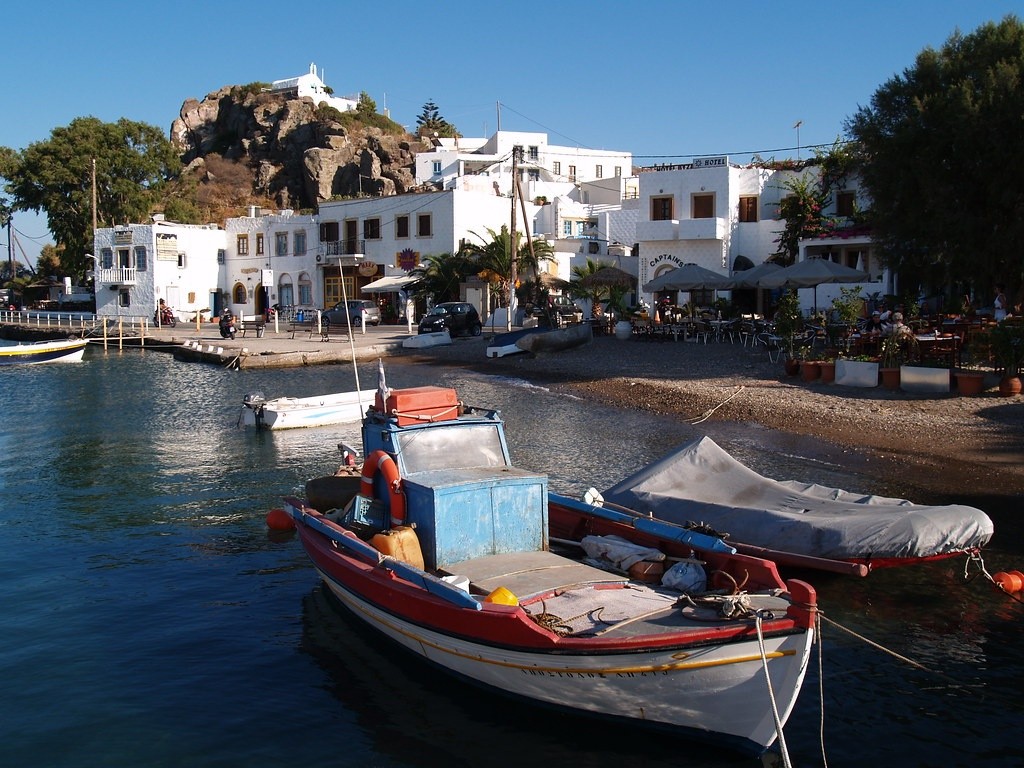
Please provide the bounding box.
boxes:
[798,360,984,394]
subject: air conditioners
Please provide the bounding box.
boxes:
[315,253,328,264]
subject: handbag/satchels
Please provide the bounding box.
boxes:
[661,554,705,594]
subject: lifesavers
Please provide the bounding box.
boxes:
[361,449,404,530]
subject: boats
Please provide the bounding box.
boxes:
[0,339,90,366]
[603,437,995,577]
[283,257,819,748]
[239,388,396,430]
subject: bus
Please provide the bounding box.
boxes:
[0,289,23,316]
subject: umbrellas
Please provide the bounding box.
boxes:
[377,358,391,414]
[725,256,789,289]
[759,255,869,323]
[642,263,728,322]
[26,276,65,299]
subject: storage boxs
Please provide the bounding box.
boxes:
[375,386,459,426]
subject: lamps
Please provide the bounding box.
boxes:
[85,254,98,260]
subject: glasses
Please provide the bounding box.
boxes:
[878,306,884,308]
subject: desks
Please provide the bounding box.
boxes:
[915,333,962,369]
[942,320,982,345]
[580,319,601,325]
[710,320,730,342]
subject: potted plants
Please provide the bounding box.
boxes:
[831,284,864,356]
[770,285,806,375]
[991,317,1024,396]
[537,196,546,206]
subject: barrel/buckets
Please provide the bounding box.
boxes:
[484,587,518,606]
[372,525,424,572]
[441,576,470,595]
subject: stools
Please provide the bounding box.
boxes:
[567,322,609,337]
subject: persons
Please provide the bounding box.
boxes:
[993,283,1009,327]
[659,296,693,321]
[159,298,166,310]
[918,302,931,319]
[866,310,912,335]
[56,289,64,308]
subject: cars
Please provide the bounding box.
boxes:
[418,301,483,340]
[523,296,584,329]
[321,299,382,327]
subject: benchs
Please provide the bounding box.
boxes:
[286,321,315,339]
[238,321,266,338]
[320,324,356,342]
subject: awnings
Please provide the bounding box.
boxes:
[360,275,425,306]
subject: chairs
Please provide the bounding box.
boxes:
[631,311,998,369]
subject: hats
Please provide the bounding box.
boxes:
[892,313,903,321]
[872,311,880,316]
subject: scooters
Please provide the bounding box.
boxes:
[153,307,176,328]
[219,308,237,340]
